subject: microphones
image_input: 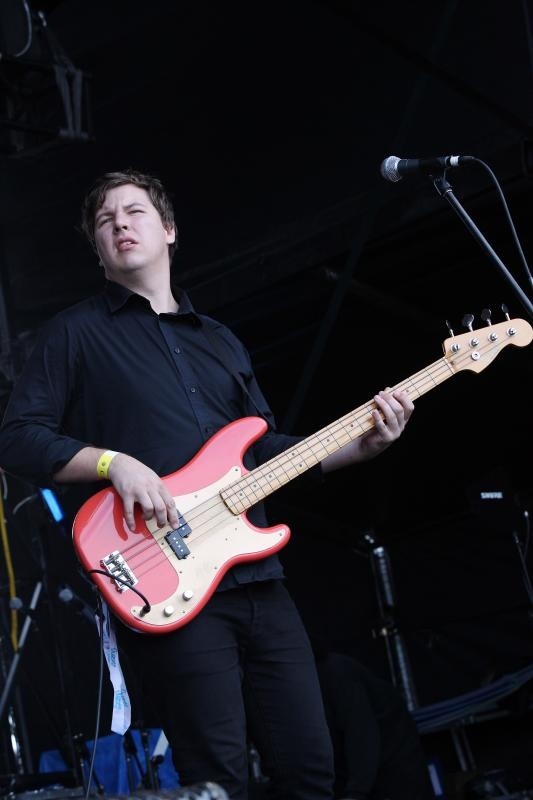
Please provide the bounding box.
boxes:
[381,155,473,182]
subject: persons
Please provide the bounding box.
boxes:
[0,169,415,800]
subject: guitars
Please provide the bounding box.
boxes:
[72,303,533,634]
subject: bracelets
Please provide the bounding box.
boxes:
[98,449,121,479]
